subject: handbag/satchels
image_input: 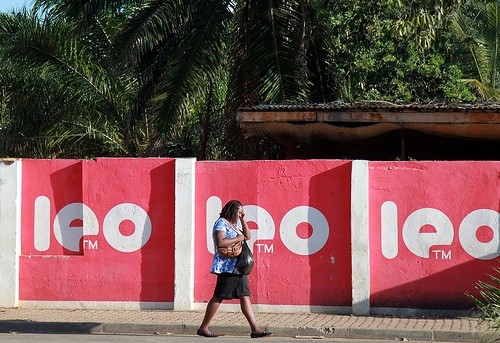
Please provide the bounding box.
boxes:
[217,240,242,258]
[234,243,254,275]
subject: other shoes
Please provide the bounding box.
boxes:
[251,330,273,338]
[197,329,218,337]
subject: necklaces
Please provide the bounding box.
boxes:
[227,220,239,233]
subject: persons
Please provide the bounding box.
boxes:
[197,200,273,338]
